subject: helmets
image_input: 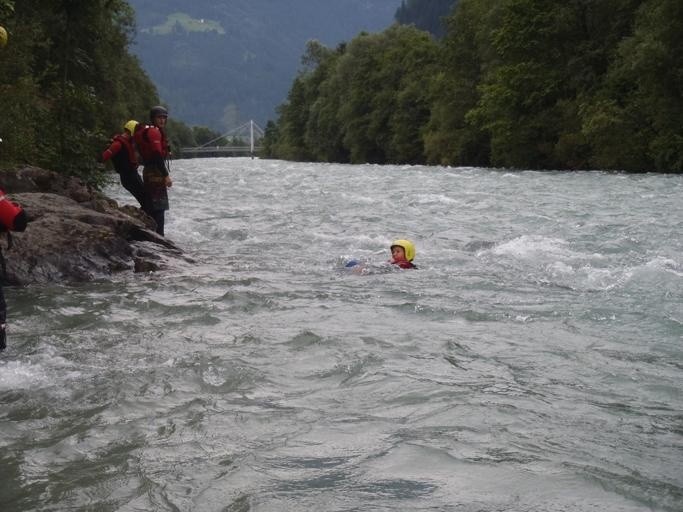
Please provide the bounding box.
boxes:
[390,239,416,262]
[124,120,139,136]
[150,106,168,123]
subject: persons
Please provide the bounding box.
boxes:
[388,240,417,269]
[102,119,144,208]
[134,106,172,237]
[0,192,27,350]
